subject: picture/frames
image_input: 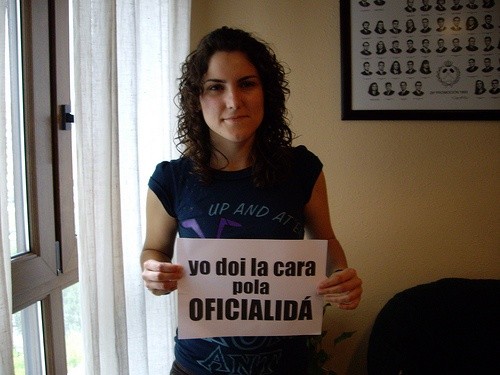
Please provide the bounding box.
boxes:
[339,2,500,122]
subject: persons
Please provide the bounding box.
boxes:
[141,26,363,374]
[358,0,500,97]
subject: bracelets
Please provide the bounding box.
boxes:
[333,269,342,273]
